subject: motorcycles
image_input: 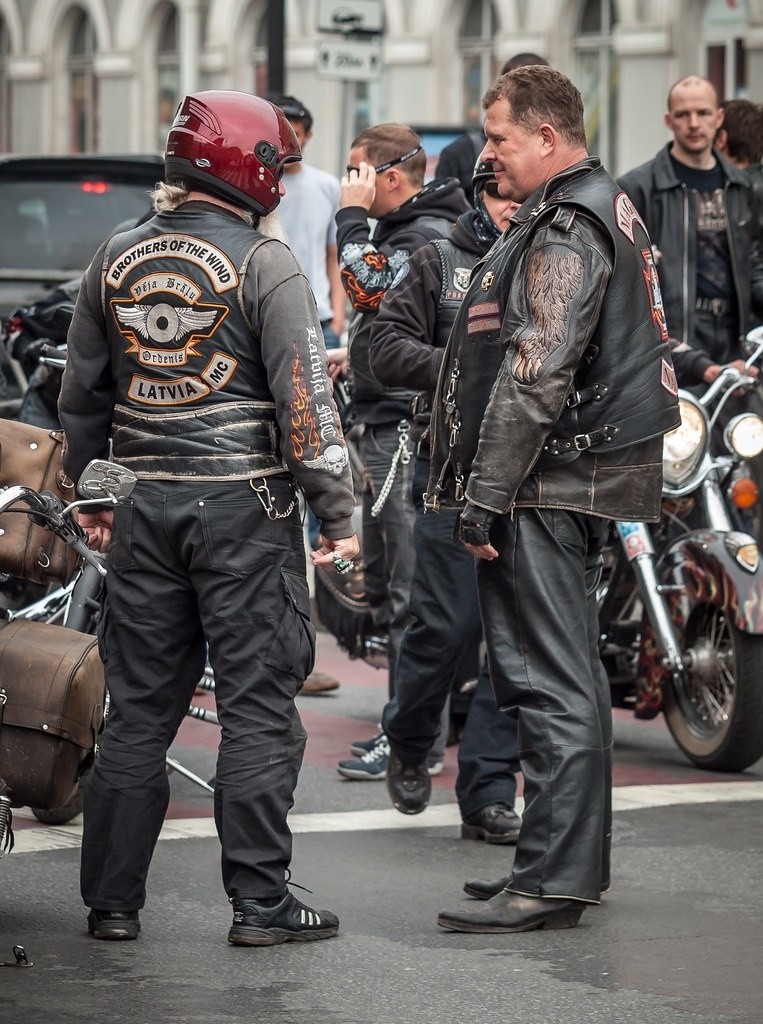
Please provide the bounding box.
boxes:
[351,325,763,773]
[0,310,221,968]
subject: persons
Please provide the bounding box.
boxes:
[59,90,360,947]
[248,51,763,844]
[437,63,679,930]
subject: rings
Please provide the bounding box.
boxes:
[330,552,354,578]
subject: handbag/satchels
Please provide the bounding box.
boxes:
[0,616,107,811]
[0,416,80,583]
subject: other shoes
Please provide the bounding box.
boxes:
[298,670,340,693]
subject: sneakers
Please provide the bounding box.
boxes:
[87,908,142,941]
[337,741,445,780]
[227,868,339,946]
[350,723,385,755]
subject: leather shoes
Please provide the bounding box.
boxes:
[437,889,587,934]
[386,748,431,814]
[462,874,512,901]
[461,802,522,845]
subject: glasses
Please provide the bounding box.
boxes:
[347,164,359,183]
[483,181,503,200]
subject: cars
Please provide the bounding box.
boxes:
[0,151,167,316]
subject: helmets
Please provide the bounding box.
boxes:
[471,148,496,186]
[165,91,303,218]
